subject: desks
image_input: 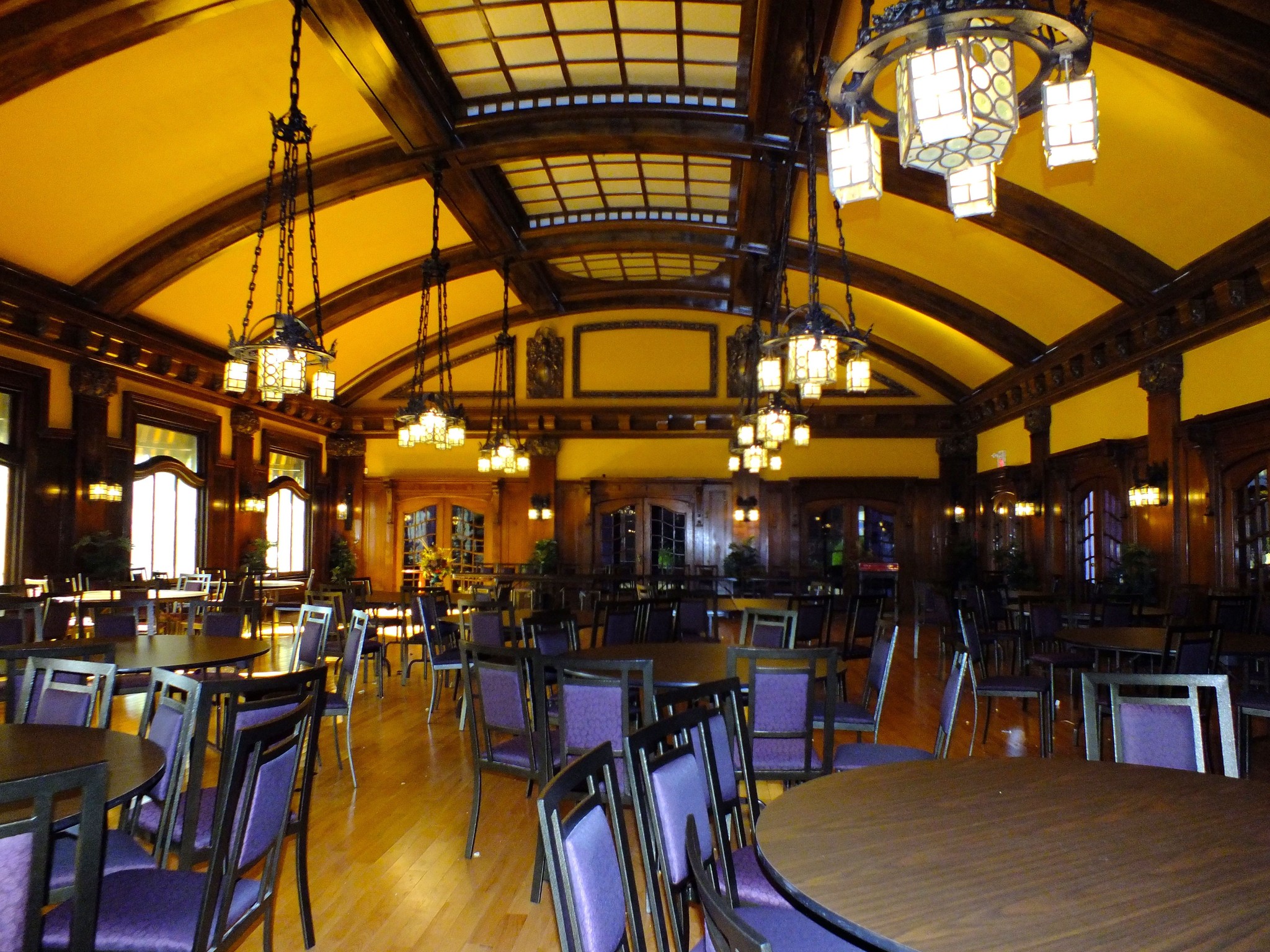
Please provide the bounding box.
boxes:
[0,636,271,678]
[67,588,209,604]
[558,642,846,691]
[751,756,1270,952]
[1051,627,1270,658]
[705,597,802,611]
[1005,603,1172,618]
[1,722,167,831]
[439,608,609,631]
[204,579,304,589]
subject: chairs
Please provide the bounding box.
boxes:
[0,565,1269,951]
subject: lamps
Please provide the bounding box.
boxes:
[728,91,870,476]
[478,255,529,472]
[529,494,552,520]
[955,474,1168,523]
[224,0,336,401]
[734,495,760,521]
[88,479,349,519]
[823,0,1102,219]
[394,156,467,449]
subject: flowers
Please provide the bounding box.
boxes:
[418,537,458,580]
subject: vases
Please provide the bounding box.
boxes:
[431,573,443,587]
